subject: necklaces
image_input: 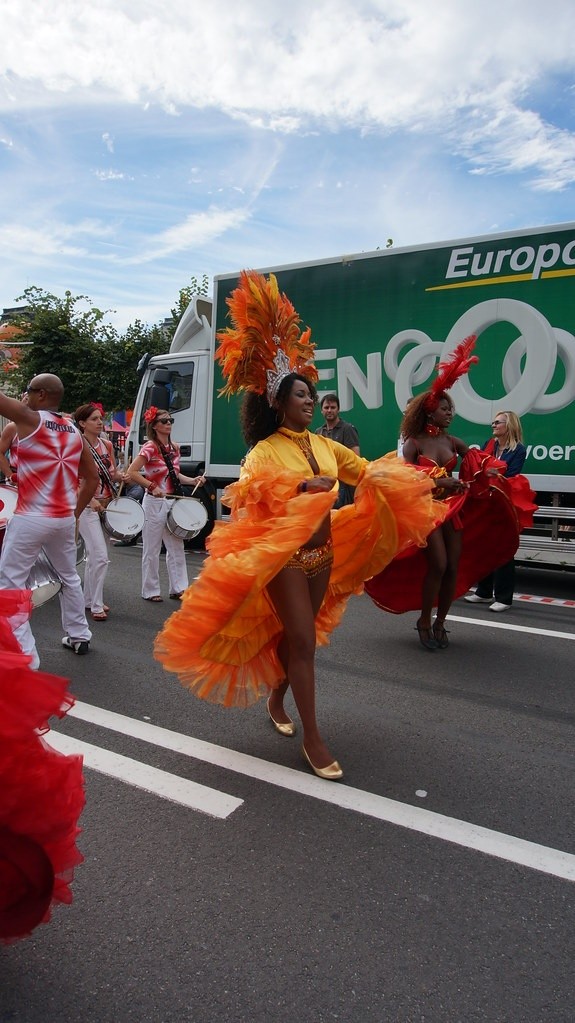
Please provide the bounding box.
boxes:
[278,424,313,461]
[424,423,444,437]
[497,446,507,452]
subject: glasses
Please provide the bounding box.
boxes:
[154,418,175,425]
[27,385,40,393]
[491,420,507,426]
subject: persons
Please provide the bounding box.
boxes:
[357,389,538,650]
[460,411,527,613]
[312,394,361,510]
[154,373,465,778]
[127,408,206,601]
[0,374,92,674]
[71,404,131,621]
[0,391,31,492]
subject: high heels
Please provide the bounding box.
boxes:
[266,697,295,736]
[414,617,438,650]
[431,623,451,648]
[299,735,343,778]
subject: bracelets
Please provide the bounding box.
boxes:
[302,481,307,492]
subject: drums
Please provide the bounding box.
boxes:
[0,484,19,549]
[24,548,64,609]
[101,496,146,541]
[76,533,87,566]
[166,498,208,540]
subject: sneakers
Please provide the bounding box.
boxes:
[488,602,511,611]
[59,636,89,656]
[465,595,492,604]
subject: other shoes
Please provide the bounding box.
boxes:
[94,604,110,620]
[171,592,184,599]
[146,594,164,602]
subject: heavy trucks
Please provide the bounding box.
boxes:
[122,222,574,568]
